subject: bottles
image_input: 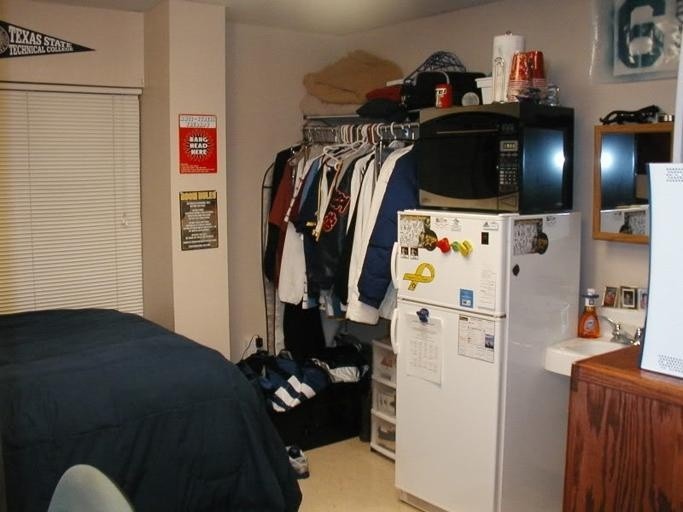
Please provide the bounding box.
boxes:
[548,82,560,106]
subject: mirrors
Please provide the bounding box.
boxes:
[593,123,675,243]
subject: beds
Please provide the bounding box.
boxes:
[0,307,302,512]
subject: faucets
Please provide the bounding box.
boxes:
[596,314,621,340]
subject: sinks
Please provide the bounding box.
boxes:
[544,335,631,378]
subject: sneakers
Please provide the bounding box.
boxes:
[284,444,310,479]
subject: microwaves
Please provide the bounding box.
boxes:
[415,105,573,211]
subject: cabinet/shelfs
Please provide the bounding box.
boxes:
[370,338,397,462]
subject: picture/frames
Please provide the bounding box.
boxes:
[637,288,648,310]
[621,286,637,309]
[601,285,620,308]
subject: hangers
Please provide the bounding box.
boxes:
[288,120,415,167]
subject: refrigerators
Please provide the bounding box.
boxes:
[601,204,650,237]
[391,208,580,512]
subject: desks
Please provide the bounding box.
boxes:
[562,346,683,512]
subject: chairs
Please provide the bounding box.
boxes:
[48,465,134,512]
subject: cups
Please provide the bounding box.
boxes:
[507,50,551,104]
[434,84,452,107]
